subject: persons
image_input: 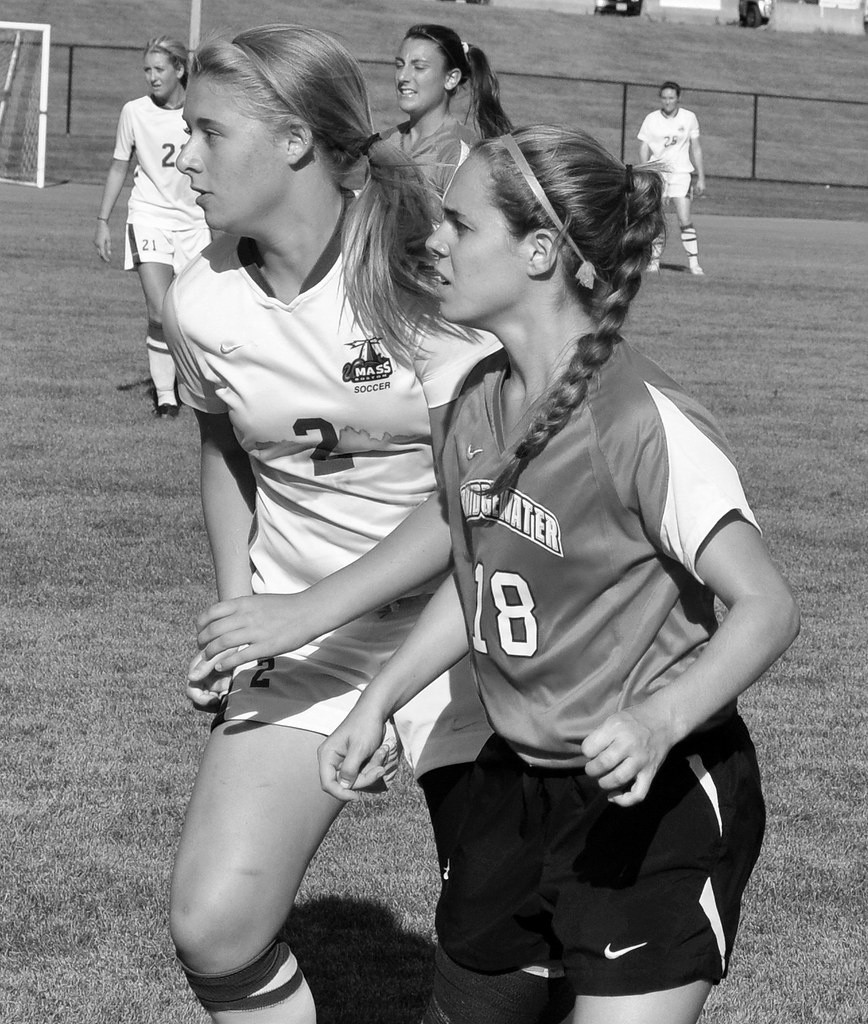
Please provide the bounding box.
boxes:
[637,80,706,274]
[317,118,800,1024]
[164,24,504,1024]
[94,37,214,419]
[376,24,513,230]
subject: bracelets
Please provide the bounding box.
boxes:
[97,217,108,223]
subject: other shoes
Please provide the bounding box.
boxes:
[157,390,179,417]
[646,261,659,271]
[690,265,704,274]
